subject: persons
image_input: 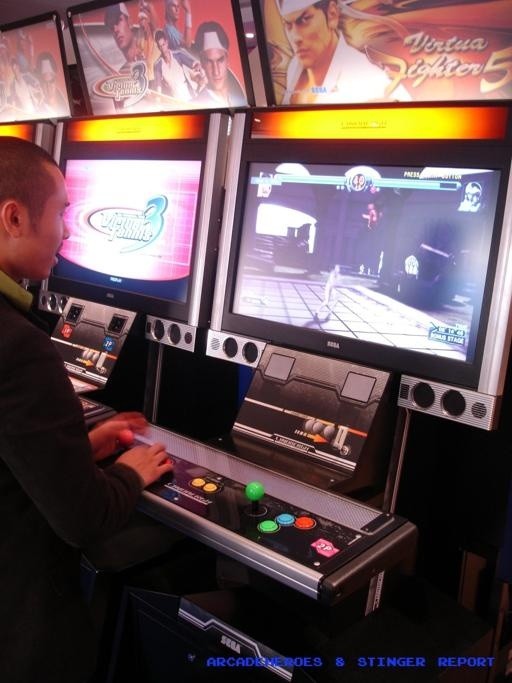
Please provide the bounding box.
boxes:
[1,1,412,120]
[0,135,175,683]
[320,265,342,318]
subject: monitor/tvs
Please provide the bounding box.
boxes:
[50,157,204,304]
[229,158,505,366]
